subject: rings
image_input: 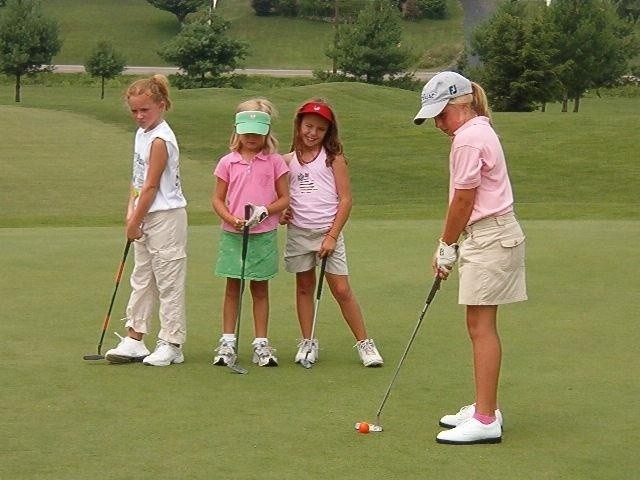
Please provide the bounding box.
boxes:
[236,219,239,224]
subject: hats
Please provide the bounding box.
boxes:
[413,71,474,126]
[296,102,333,123]
[234,111,271,136]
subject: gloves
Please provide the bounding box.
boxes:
[240,202,269,231]
[436,239,459,273]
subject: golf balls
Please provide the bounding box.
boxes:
[359,424,368,432]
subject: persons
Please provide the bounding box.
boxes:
[102,75,187,366]
[280,97,383,368]
[211,98,291,369]
[408,69,528,443]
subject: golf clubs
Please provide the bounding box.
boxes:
[228,205,251,375]
[302,253,326,368]
[83,237,131,360]
[355,245,457,433]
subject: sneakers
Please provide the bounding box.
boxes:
[439,402,503,433]
[212,337,238,367]
[251,340,278,367]
[294,338,319,364]
[142,340,185,367]
[104,331,151,364]
[436,417,502,445]
[353,338,383,367]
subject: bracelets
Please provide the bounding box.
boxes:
[327,233,337,242]
